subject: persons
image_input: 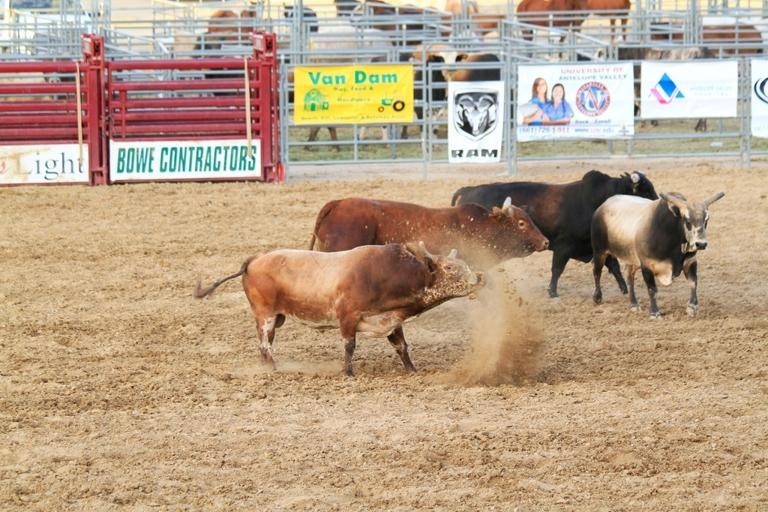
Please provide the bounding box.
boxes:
[520,78,552,126]
[540,82,575,125]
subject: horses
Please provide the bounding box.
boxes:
[516,0,631,60]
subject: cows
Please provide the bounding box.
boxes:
[589,191,725,322]
[191,0,505,151]
[194,240,488,381]
[308,196,550,271]
[451,169,660,299]
